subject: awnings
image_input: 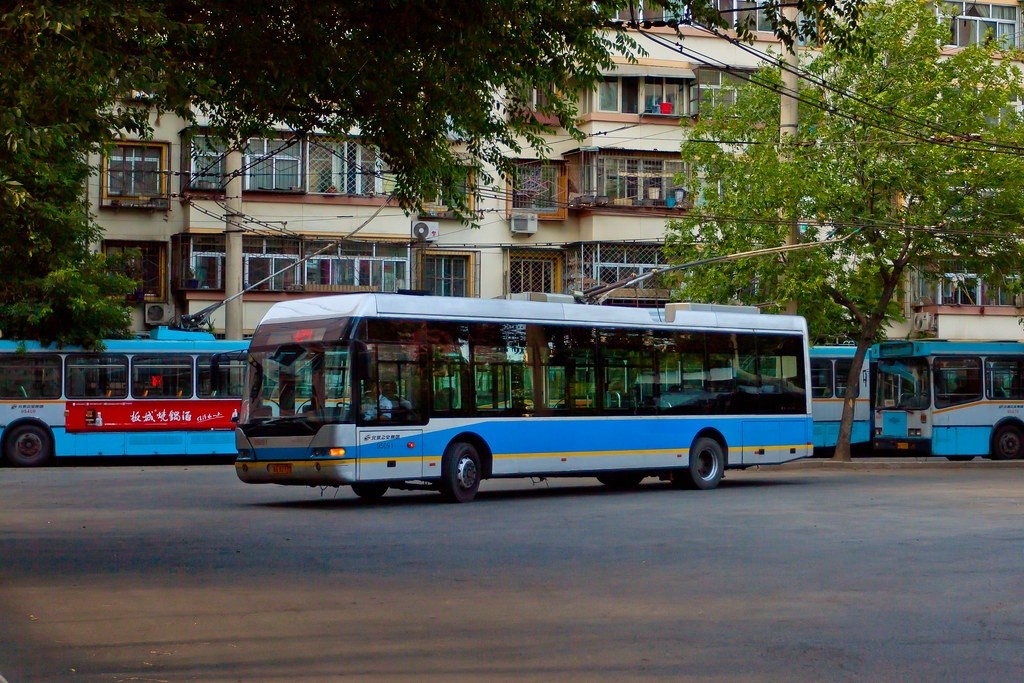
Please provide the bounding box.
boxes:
[585,61,696,79]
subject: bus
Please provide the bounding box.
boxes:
[739,344,872,448]
[872,338,1024,460]
[1,325,355,467]
[233,291,815,506]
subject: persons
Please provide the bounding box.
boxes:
[362,383,391,420]
[995,375,1006,398]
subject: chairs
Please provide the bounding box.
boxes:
[632,364,736,413]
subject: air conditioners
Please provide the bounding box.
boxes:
[145,303,176,325]
[912,312,935,332]
[510,212,537,234]
[411,221,440,240]
